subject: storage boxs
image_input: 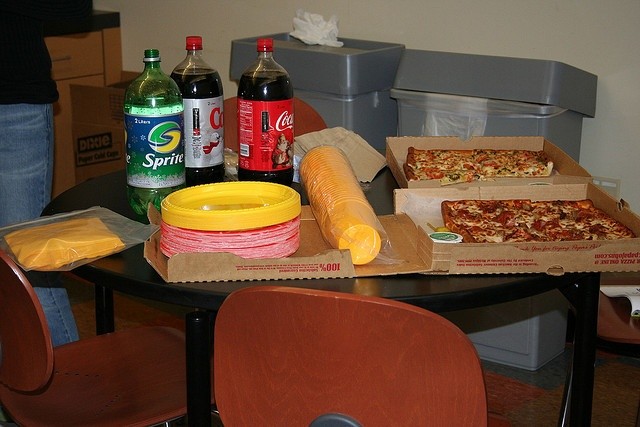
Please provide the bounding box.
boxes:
[430,275,569,371]
[69,70,144,186]
[388,48,598,165]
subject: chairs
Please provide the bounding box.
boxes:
[214,284,489,426]
[0,250,214,427]
[43,10,124,202]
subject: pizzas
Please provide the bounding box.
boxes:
[402,146,554,186]
[440,199,637,245]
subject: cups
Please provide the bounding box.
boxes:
[298,144,382,266]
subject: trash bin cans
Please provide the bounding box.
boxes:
[389,48,597,372]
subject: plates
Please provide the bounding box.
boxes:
[160,180,302,260]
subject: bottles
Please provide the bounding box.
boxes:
[124,48,186,216]
[169,34,225,188]
[236,37,295,187]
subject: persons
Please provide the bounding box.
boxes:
[0,1,94,348]
